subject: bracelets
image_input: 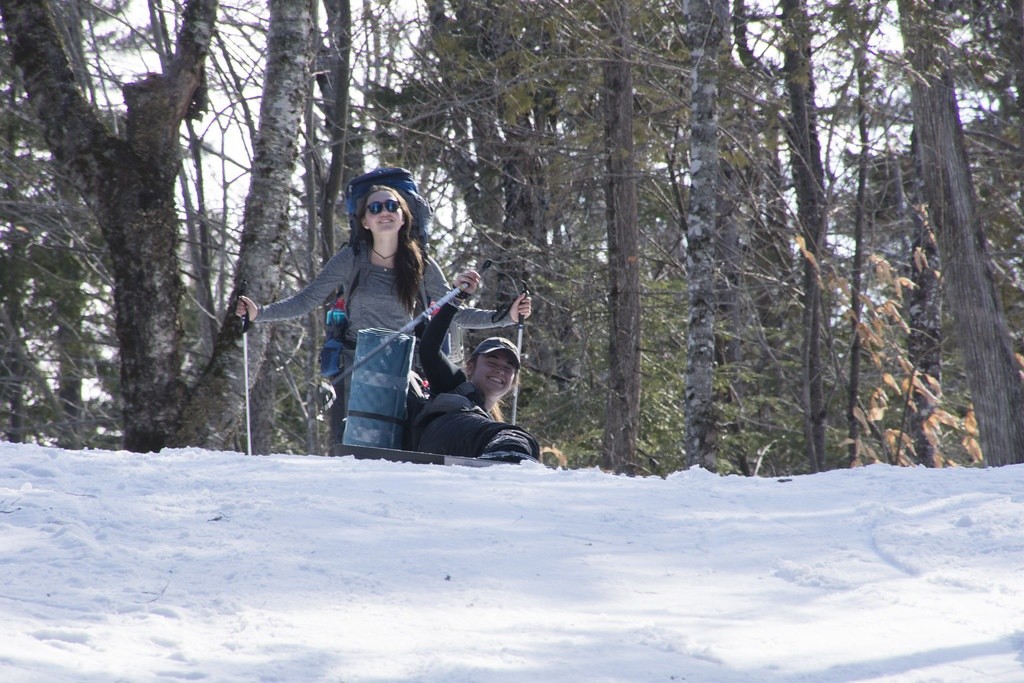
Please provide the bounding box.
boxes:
[450,296,462,306]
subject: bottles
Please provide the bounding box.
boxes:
[326,295,346,329]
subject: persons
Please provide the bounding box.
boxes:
[235,184,532,442]
[409,268,541,463]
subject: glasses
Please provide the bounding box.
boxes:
[366,199,400,215]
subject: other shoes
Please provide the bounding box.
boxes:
[477,441,540,466]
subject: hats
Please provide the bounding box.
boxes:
[471,336,521,369]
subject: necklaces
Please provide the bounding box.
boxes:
[372,248,399,271]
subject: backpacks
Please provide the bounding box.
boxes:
[319,167,452,377]
